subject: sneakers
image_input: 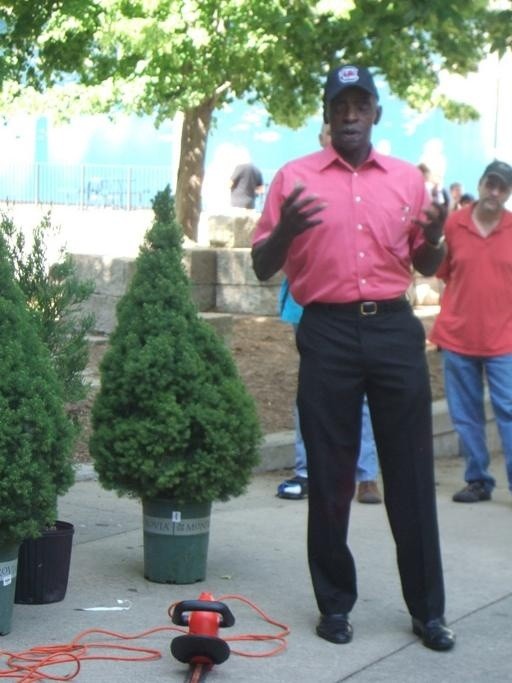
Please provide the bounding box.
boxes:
[277,475,309,500]
[358,480,381,504]
[453,479,490,503]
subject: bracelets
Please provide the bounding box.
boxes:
[422,232,448,253]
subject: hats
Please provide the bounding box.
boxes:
[483,159,511,185]
[323,64,378,104]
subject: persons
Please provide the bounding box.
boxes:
[277,121,383,507]
[230,166,265,211]
[416,160,512,504]
[249,63,456,650]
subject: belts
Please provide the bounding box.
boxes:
[348,300,406,315]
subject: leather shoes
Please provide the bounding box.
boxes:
[316,614,353,642]
[411,615,455,650]
